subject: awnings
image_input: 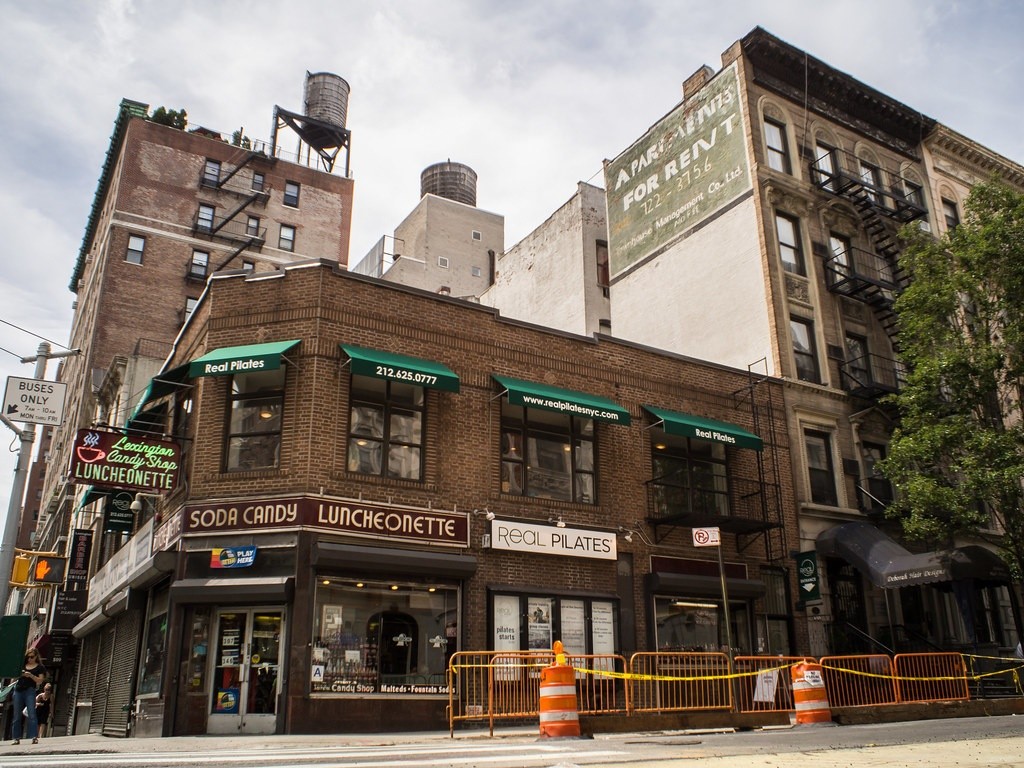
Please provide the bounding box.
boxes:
[133,363,190,421]
[818,522,1010,586]
[124,402,166,435]
[74,486,108,518]
[338,344,460,393]
[490,372,630,425]
[0,615,31,678]
[0,685,14,703]
[188,339,301,376]
[29,635,49,649]
[643,404,763,450]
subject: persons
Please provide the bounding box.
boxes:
[1016,642,1024,684]
[36,682,54,737]
[11,647,46,745]
[537,607,543,622]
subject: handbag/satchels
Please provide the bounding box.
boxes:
[0,680,18,702]
[22,706,29,717]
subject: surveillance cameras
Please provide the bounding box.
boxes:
[130,500,142,514]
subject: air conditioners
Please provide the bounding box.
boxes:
[910,220,931,232]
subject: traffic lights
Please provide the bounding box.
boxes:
[32,556,69,585]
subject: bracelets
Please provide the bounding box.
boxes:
[30,675,33,678]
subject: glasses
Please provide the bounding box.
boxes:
[26,656,35,659]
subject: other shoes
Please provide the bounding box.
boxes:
[11,738,20,745]
[32,737,38,744]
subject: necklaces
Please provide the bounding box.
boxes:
[45,698,46,699]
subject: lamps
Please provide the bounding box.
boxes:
[483,508,495,522]
[556,516,565,528]
[624,532,634,543]
